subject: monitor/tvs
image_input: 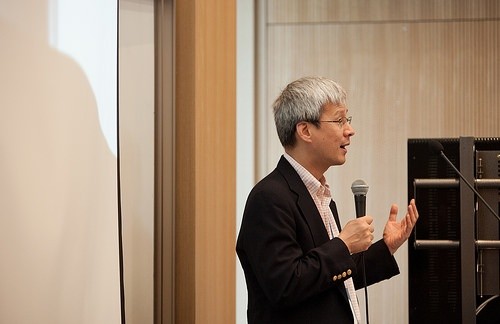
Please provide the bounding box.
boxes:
[407,136,500,324]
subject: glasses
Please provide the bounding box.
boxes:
[314,117,352,128]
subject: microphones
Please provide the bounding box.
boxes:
[431,141,500,221]
[350,178,369,253]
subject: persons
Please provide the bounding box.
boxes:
[236,76,419,324]
